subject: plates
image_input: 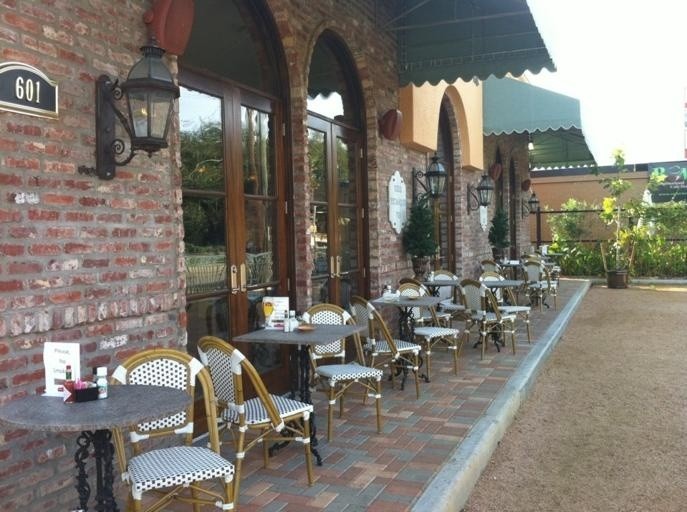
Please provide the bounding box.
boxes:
[408,295,420,300]
[297,326,315,331]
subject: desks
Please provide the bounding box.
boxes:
[232,325,367,465]
[372,295,442,390]
[1,385,192,509]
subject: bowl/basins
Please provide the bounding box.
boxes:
[75,386,97,403]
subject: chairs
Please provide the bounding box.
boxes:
[107,349,236,512]
[395,282,459,379]
[396,247,562,359]
[349,294,424,401]
[300,304,384,442]
[195,336,316,500]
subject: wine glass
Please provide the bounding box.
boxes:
[262,300,274,328]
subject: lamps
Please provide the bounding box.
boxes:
[93,33,180,179]
[521,191,539,221]
[411,149,448,210]
[467,170,495,215]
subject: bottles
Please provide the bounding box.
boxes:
[283,310,295,333]
[90,365,109,400]
[430,271,434,281]
[383,289,387,298]
[386,285,391,297]
[62,364,76,405]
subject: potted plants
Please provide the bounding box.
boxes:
[599,145,644,288]
[395,194,439,280]
[485,204,512,261]
